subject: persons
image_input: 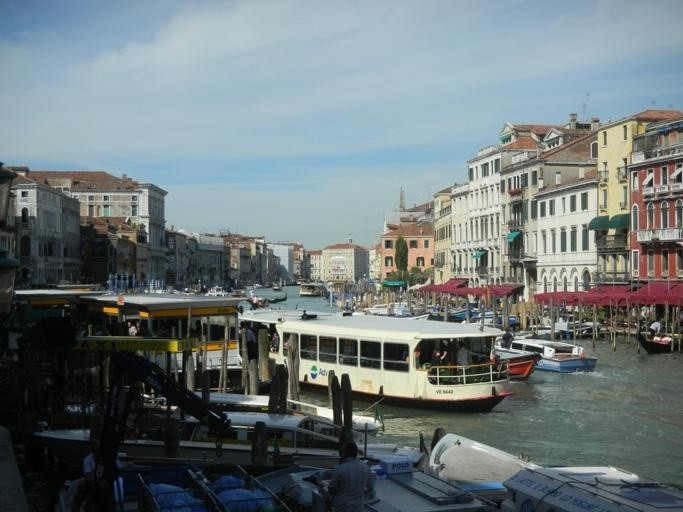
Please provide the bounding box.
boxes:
[252,295,257,311]
[647,319,665,340]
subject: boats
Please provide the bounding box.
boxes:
[636,330,679,356]
[300,284,323,297]
[4,288,248,393]
